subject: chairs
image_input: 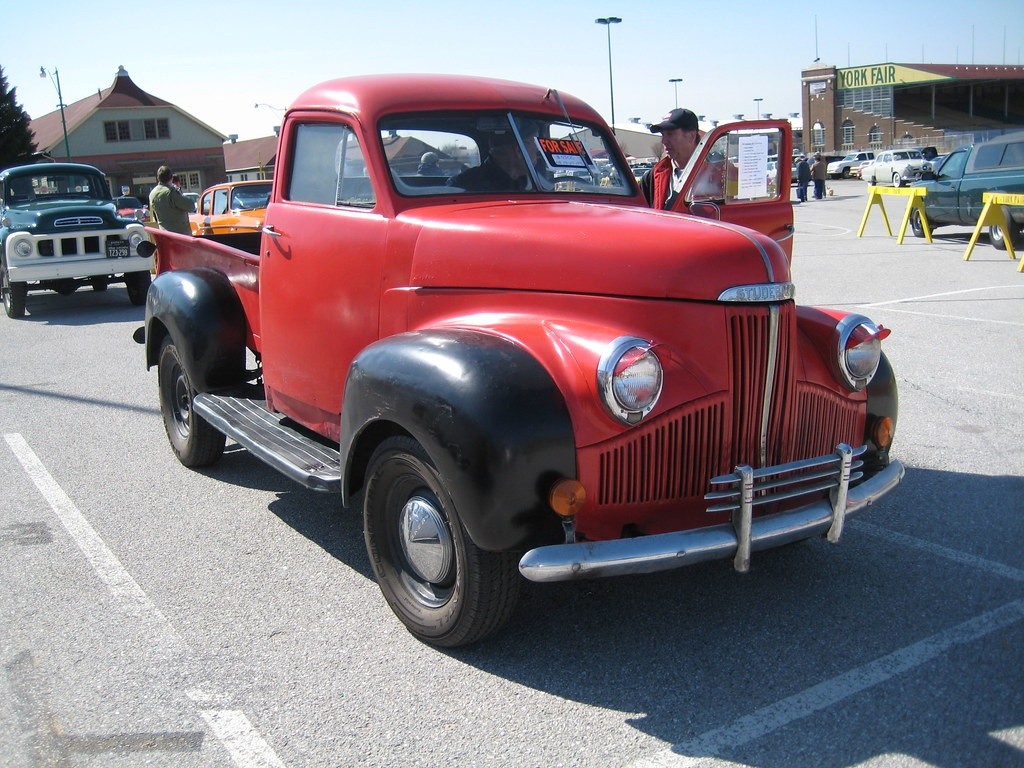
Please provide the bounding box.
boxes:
[14,183,35,199]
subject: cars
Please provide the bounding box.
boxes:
[187,179,275,237]
[768,146,946,188]
[552,158,658,193]
[112,190,200,222]
[0,162,156,319]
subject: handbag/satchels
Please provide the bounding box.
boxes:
[796,182,804,199]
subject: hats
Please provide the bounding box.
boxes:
[488,118,540,146]
[420,152,439,166]
[650,108,699,133]
[795,156,801,164]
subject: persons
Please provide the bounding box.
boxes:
[794,157,810,202]
[418,152,444,176]
[810,155,825,199]
[637,108,737,208]
[444,117,542,192]
[152,165,195,236]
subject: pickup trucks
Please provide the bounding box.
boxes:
[134,75,907,653]
[909,131,1024,250]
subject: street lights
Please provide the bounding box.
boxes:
[39,66,72,163]
[753,99,763,120]
[593,17,622,132]
[668,78,683,110]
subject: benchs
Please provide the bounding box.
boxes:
[335,174,452,202]
